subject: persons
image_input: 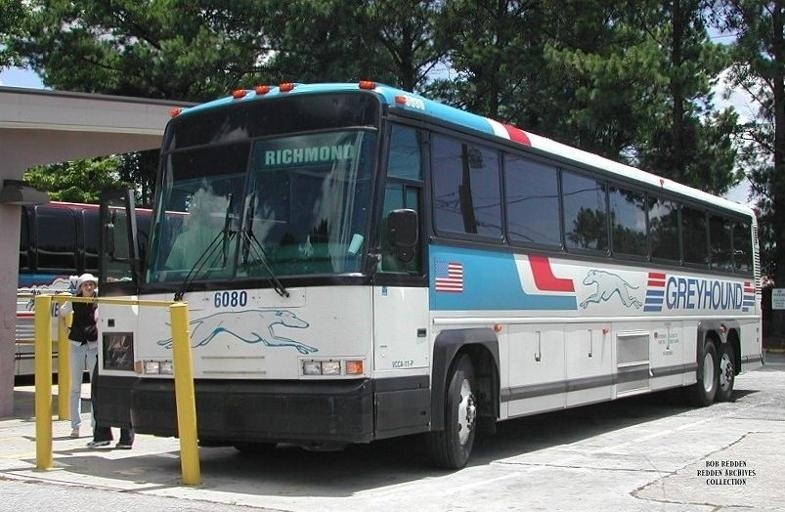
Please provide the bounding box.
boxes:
[58,273,99,438]
[86,276,135,450]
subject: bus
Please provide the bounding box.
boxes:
[95,82,762,471]
[13,199,288,389]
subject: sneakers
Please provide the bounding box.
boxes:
[71,429,79,437]
[116,443,131,448]
[88,440,109,447]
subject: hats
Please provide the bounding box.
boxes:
[77,273,98,288]
